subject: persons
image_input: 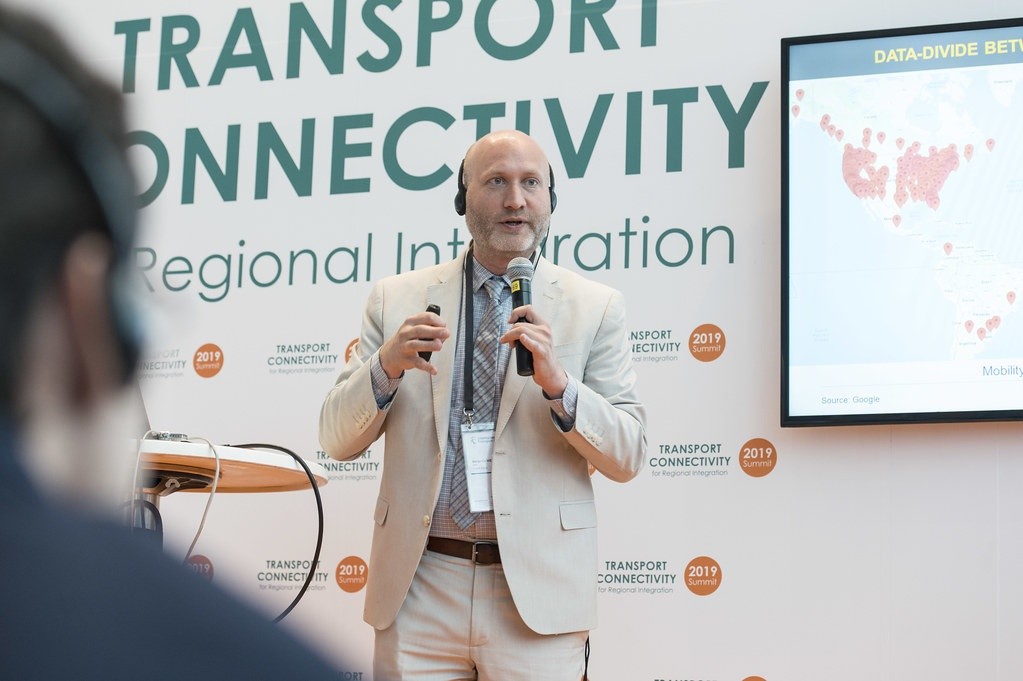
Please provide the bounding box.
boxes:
[318,129,647,681]
[0,0,363,681]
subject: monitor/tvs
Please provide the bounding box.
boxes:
[779,17,1023,428]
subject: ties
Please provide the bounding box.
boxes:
[448,279,510,531]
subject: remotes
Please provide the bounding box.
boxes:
[418,305,440,362]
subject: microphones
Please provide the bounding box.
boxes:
[508,257,536,376]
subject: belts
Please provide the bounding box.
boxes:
[426,536,501,565]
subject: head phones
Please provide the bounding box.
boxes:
[454,159,557,216]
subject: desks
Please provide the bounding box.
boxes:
[122,439,329,534]
